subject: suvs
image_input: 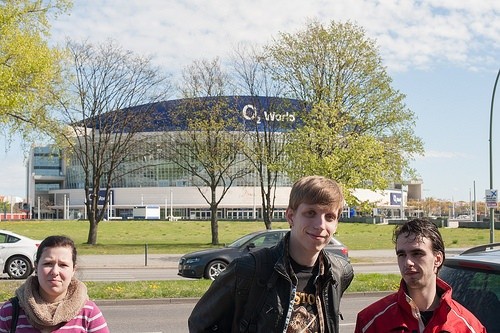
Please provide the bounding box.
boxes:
[177,228,351,280]
[437,242,500,333]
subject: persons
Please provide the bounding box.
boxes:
[188,175,354,333]
[0,236,110,333]
[354,218,486,333]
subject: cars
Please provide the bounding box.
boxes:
[0,229,42,279]
